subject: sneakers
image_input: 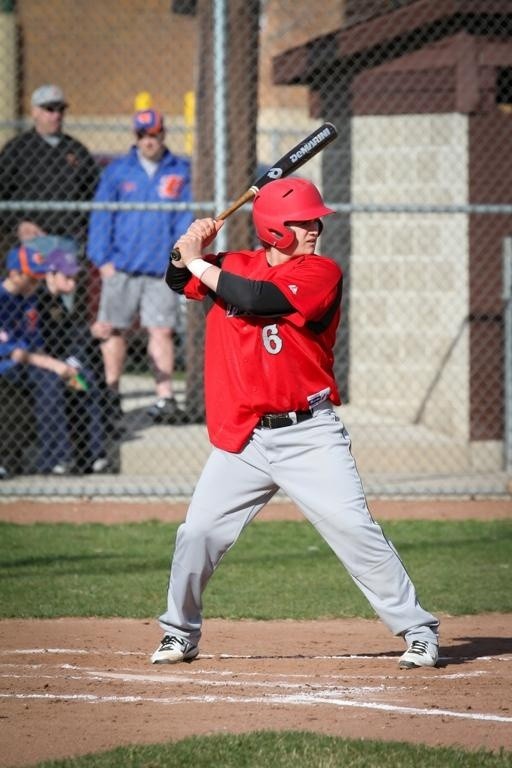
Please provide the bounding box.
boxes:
[0,457,116,478]
[398,638,439,670]
[148,397,179,421]
[151,632,200,665]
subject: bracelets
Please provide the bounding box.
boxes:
[187,258,212,280]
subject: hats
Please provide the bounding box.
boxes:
[31,84,70,108]
[6,246,55,281]
[44,249,84,280]
[132,109,165,136]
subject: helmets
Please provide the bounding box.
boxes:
[251,176,340,251]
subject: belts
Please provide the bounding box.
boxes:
[256,409,312,429]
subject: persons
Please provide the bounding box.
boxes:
[85,107,195,416]
[149,179,442,668]
[0,83,119,480]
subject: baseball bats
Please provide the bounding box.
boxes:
[170,121,339,260]
